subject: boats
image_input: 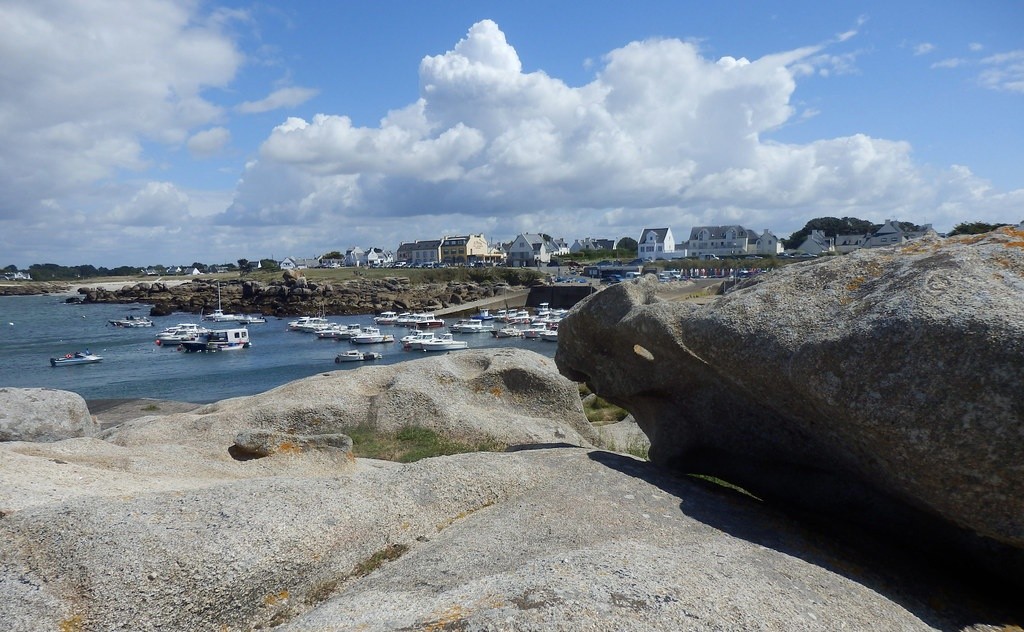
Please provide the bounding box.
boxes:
[350,327,395,343]
[448,319,494,333]
[470,309,535,324]
[50,348,103,367]
[373,312,398,325]
[399,330,468,350]
[155,324,249,351]
[332,323,362,339]
[489,321,559,341]
[108,315,154,327]
[205,310,266,324]
[530,303,568,322]
[336,350,378,361]
[288,316,347,338]
[393,313,445,328]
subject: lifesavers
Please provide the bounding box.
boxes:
[66,354,71,358]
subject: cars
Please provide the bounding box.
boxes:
[555,269,682,283]
[370,262,448,269]
[496,261,512,267]
[317,262,345,269]
[596,253,817,267]
